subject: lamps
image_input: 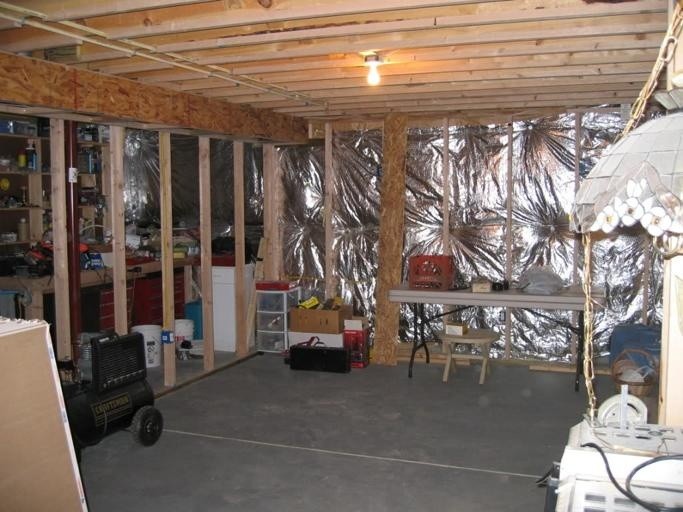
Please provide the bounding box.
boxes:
[363,54,381,86]
[569,85,682,264]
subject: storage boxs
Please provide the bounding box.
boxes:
[287,297,371,373]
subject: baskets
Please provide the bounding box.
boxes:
[611,349,656,399]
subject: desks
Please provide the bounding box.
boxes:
[1,257,201,319]
[389,286,606,390]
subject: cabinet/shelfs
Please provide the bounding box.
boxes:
[0,135,113,246]
[254,287,298,357]
[212,262,256,353]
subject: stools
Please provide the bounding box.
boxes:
[439,329,501,385]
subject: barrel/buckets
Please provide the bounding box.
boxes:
[130,325,161,368]
[175,319,194,347]
